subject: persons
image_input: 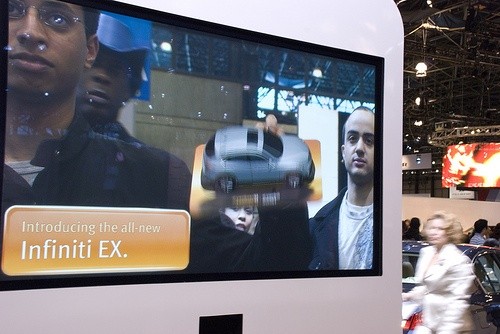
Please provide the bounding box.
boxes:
[221,201,259,234]
[78,8,153,146]
[469,219,488,246]
[304,106,374,270]
[403,210,476,334]
[0,0,310,281]
[485,222,500,247]
[402,217,424,240]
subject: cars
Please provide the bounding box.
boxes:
[402,238,499,334]
[200,125,316,196]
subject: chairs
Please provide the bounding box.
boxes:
[402,240,500,328]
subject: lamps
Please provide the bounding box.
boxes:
[414,30,427,127]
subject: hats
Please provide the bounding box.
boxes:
[96,10,152,101]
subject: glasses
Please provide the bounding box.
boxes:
[8,0,87,29]
